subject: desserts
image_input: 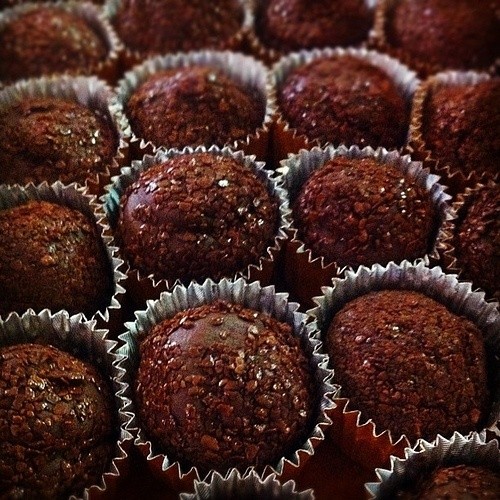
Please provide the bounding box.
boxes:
[0,0,500,500]
